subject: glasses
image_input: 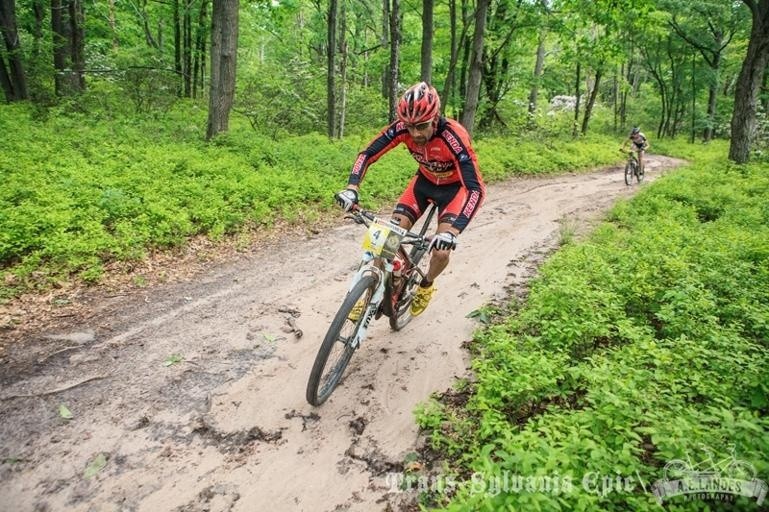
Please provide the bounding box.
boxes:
[403,122,430,132]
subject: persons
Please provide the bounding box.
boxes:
[621,127,650,181]
[336,80,487,318]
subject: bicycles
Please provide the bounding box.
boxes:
[305,192,457,407]
[619,147,645,186]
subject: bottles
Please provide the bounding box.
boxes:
[392,260,401,286]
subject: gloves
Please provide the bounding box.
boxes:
[426,230,457,255]
[333,188,360,213]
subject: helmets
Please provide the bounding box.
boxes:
[631,128,640,134]
[395,80,441,127]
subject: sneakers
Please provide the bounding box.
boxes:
[409,279,435,318]
[346,299,366,322]
